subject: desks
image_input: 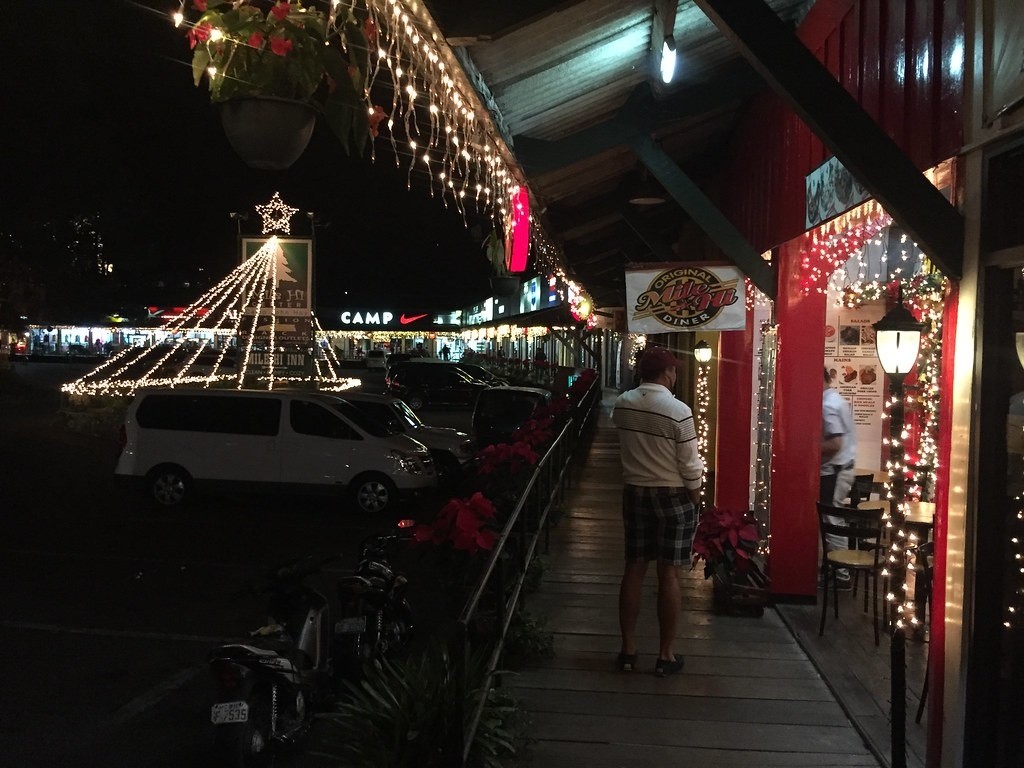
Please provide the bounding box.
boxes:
[856,500,936,643]
[854,468,893,493]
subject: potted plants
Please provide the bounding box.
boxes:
[504,611,557,662]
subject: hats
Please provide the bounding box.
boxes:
[639,346,685,368]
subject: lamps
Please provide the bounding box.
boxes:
[692,338,713,366]
[871,285,926,379]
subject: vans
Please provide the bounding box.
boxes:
[113,388,438,517]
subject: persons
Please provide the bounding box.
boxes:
[609,348,704,673]
[818,368,857,590]
[497,347,505,356]
[426,346,429,352]
[534,348,547,361]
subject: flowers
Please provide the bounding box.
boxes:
[410,354,595,554]
[689,505,764,580]
[181,0,387,157]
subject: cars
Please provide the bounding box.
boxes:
[384,361,509,390]
[385,353,411,372]
[322,391,482,490]
[471,386,553,445]
[366,351,385,369]
[390,365,490,411]
[139,349,186,381]
[15,341,26,353]
[180,355,240,384]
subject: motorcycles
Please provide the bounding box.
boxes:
[208,552,342,768]
[329,519,416,698]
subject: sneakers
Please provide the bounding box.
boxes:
[619,649,638,673]
[817,576,853,593]
[655,653,685,678]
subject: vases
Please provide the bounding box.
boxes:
[217,94,319,171]
[712,568,768,618]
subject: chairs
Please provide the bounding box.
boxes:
[816,501,891,646]
[848,473,874,551]
[916,541,933,614]
